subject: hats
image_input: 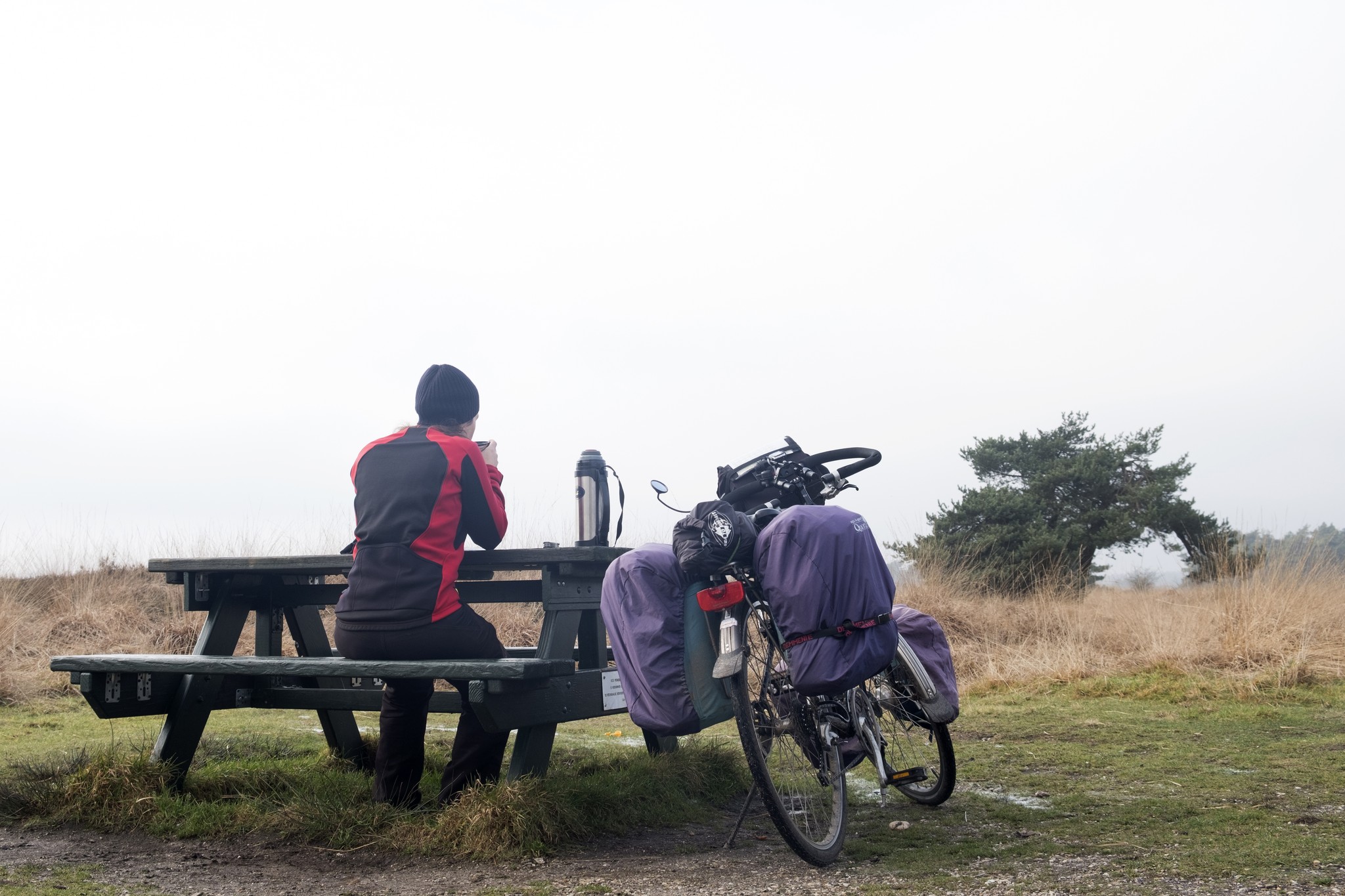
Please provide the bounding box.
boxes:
[415,364,479,425]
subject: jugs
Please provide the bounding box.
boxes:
[573,450,610,547]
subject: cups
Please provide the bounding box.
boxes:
[474,442,489,452]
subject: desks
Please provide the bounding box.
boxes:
[143,546,631,807]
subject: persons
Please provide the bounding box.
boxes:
[334,364,511,811]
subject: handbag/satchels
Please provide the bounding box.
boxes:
[885,605,960,729]
[673,499,757,579]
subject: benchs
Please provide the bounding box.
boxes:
[330,646,615,661]
[51,653,577,719]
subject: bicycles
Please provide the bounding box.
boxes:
[597,438,957,868]
